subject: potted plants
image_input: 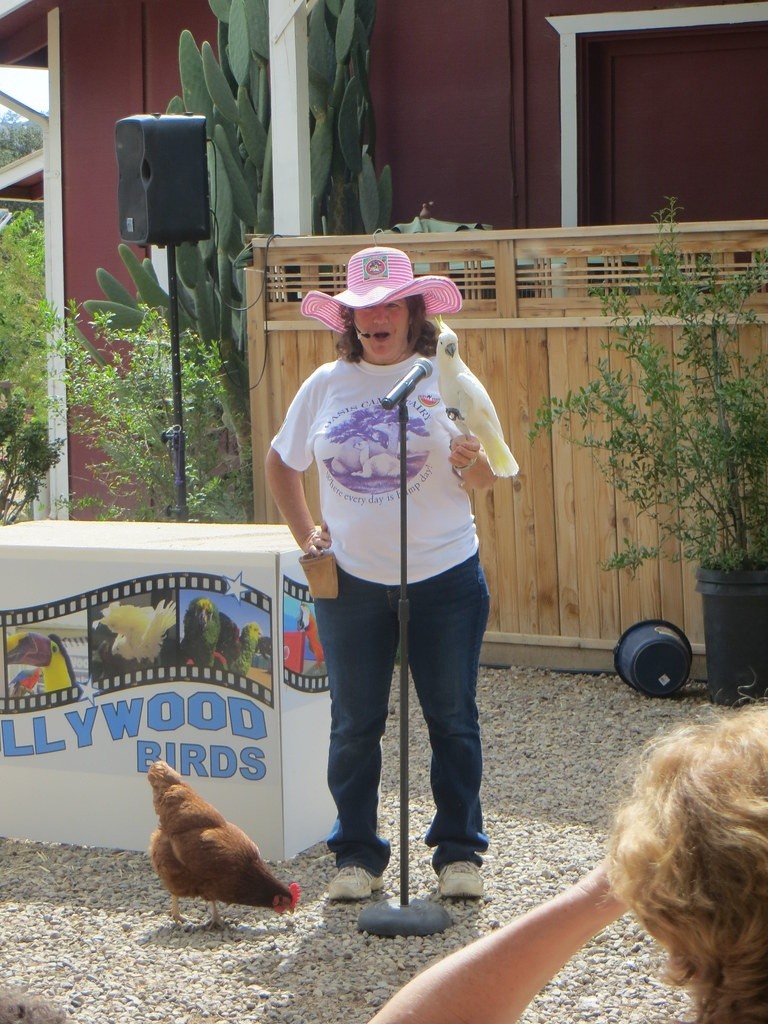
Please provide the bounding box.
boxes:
[526,193,767,708]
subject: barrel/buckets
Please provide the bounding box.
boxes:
[612,619,694,696]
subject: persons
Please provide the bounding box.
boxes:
[365,700,768,1024]
[266,245,491,900]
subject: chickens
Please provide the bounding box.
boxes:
[147,761,300,931]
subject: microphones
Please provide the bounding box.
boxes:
[352,320,370,338]
[381,358,433,410]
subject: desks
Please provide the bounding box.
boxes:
[0,521,339,864]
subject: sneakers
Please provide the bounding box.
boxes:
[440,861,482,898]
[329,865,384,898]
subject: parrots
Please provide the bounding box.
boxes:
[434,313,520,478]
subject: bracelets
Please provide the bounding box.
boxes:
[454,458,477,470]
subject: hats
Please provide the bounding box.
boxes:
[301,247,461,332]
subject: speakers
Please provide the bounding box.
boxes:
[115,112,212,247]
